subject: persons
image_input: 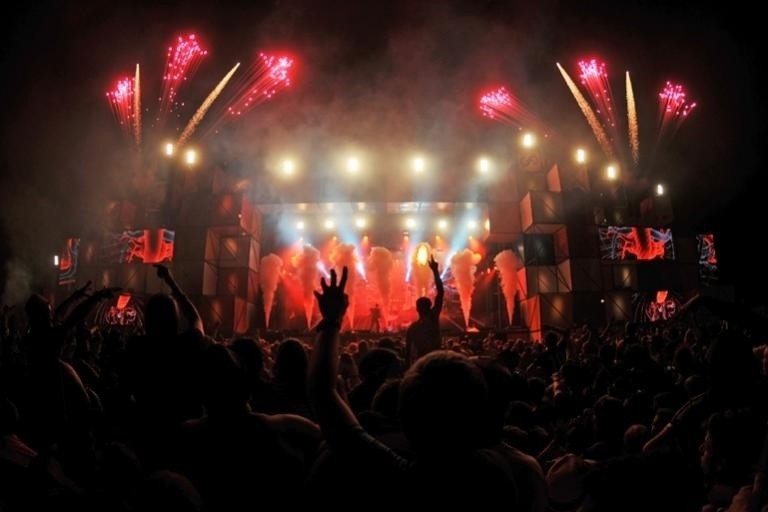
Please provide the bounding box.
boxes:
[2,256,768,511]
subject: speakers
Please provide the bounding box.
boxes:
[603,155,633,227]
[636,158,674,225]
